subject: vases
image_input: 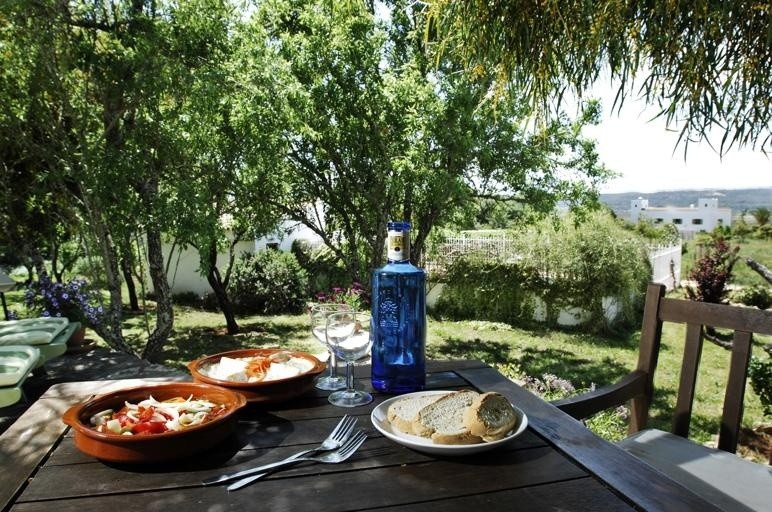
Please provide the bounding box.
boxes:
[6,266,107,324]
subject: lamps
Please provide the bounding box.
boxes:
[1,298,45,408]
[546,273,770,512]
[2,308,81,364]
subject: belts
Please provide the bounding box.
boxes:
[312,302,377,408]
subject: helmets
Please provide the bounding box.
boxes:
[370,389,529,455]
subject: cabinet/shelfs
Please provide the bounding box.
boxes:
[368,222,426,392]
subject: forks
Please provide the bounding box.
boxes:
[202,412,368,492]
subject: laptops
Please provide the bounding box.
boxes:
[388,388,519,445]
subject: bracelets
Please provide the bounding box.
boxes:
[189,348,326,398]
[61,382,247,463]
